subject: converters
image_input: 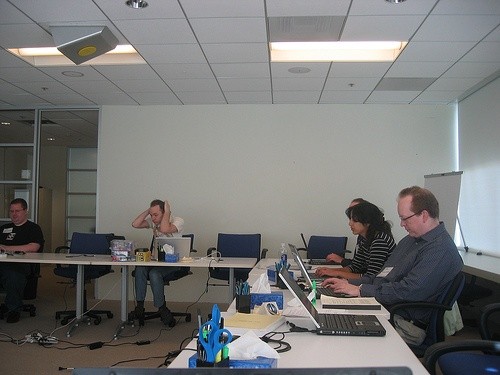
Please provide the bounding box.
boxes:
[89,341,104,350]
[136,339,151,346]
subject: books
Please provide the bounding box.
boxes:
[224,312,286,334]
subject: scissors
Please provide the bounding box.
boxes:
[199,321,232,361]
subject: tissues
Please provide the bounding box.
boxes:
[249,273,284,311]
[188,331,280,369]
[268,265,293,283]
[160,243,179,263]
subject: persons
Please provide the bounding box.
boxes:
[0,198,45,323]
[127,200,184,325]
[321,186,464,346]
[326,198,367,267]
[316,201,396,280]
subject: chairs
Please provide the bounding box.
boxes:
[53,232,114,326]
[207,233,268,321]
[297,233,351,258]
[132,234,198,327]
[388,268,467,358]
[424,302,500,375]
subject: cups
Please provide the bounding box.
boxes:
[276,270,293,289]
[158,252,165,262]
[196,356,229,368]
[236,293,250,314]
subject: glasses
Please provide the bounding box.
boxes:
[399,208,427,221]
[8,209,25,214]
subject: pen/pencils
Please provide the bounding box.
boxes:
[212,304,224,344]
[275,261,291,270]
[234,279,250,295]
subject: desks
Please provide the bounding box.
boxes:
[0,251,430,374]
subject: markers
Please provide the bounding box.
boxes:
[222,346,229,359]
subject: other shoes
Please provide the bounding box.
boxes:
[7,310,19,323]
[135,300,145,315]
[157,301,169,320]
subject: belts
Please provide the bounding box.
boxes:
[403,315,427,332]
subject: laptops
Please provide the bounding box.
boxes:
[277,266,387,337]
[290,251,358,299]
[151,236,192,261]
[287,243,331,282]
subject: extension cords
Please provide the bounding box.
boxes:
[27,335,58,343]
